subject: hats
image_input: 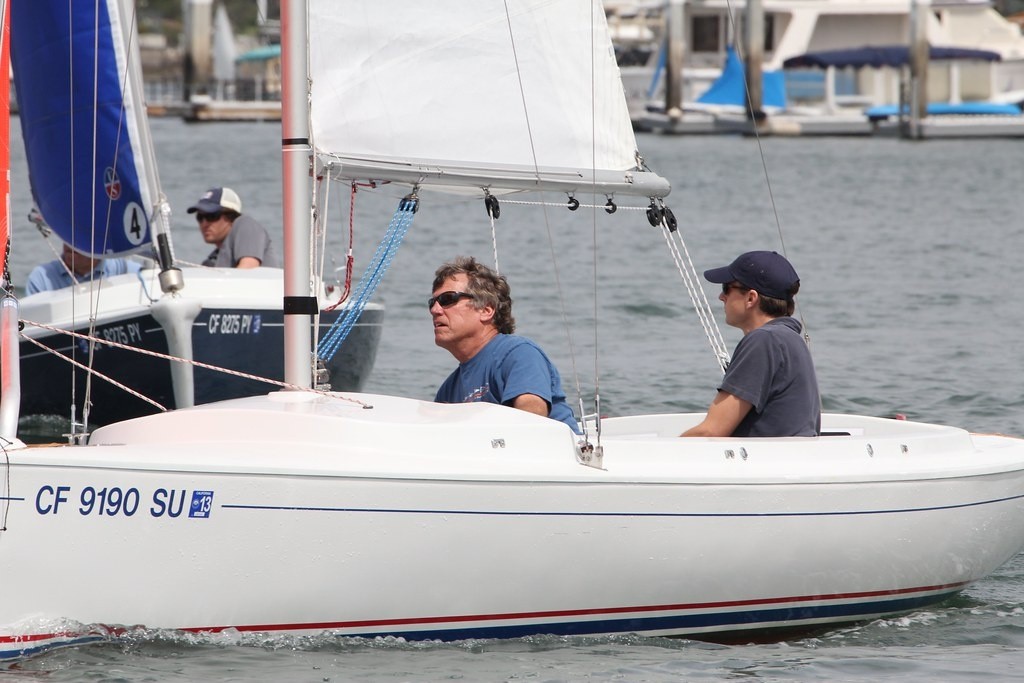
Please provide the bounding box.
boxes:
[703,250,800,300]
[187,187,242,215]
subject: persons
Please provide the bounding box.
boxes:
[187,186,280,268]
[428,256,584,435]
[678,250,821,437]
[25,242,145,296]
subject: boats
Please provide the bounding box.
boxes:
[621,0,1023,139]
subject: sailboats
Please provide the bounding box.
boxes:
[0,0,388,446]
[0,0,1023,662]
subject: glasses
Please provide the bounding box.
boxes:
[428,290,473,310]
[196,209,232,222]
[722,281,762,302]
